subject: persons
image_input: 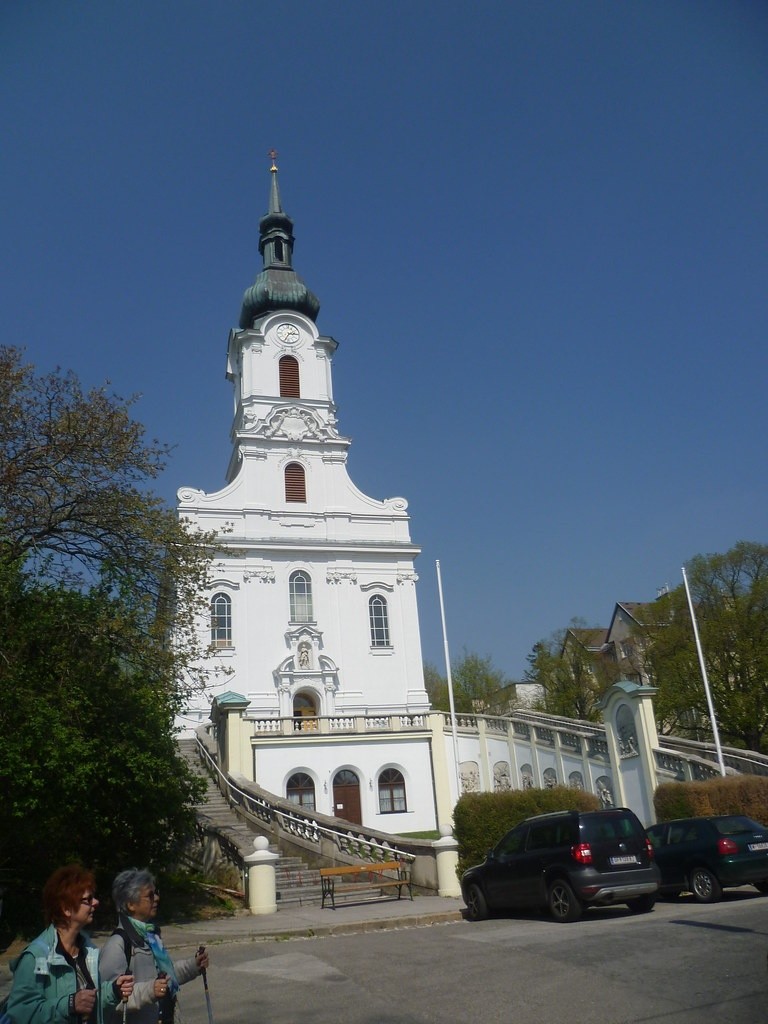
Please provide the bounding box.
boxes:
[300,644,308,666]
[97,870,209,1024]
[0,866,135,1024]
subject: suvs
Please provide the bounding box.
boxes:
[459,808,661,923]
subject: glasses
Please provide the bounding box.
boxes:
[81,894,94,903]
[139,889,159,900]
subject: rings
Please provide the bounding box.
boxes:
[161,988,163,992]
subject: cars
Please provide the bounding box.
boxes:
[645,814,767,899]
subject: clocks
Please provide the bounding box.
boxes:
[277,324,298,342]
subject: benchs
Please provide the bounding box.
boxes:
[319,862,415,910]
